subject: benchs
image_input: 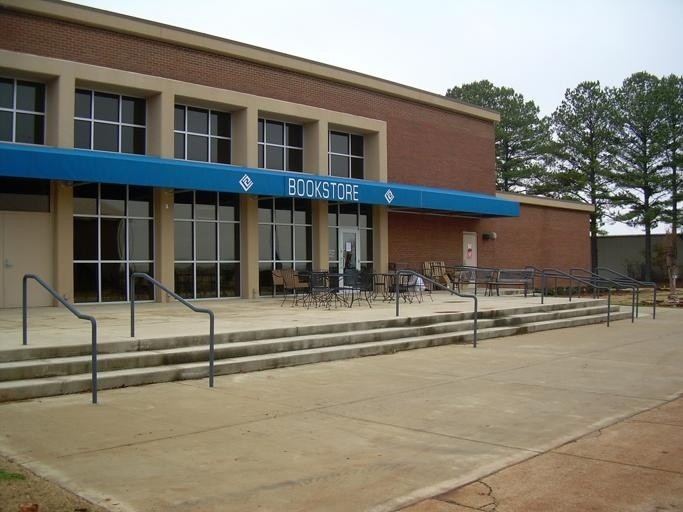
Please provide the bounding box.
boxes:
[444,262,537,298]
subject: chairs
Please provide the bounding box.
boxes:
[268,257,435,306]
